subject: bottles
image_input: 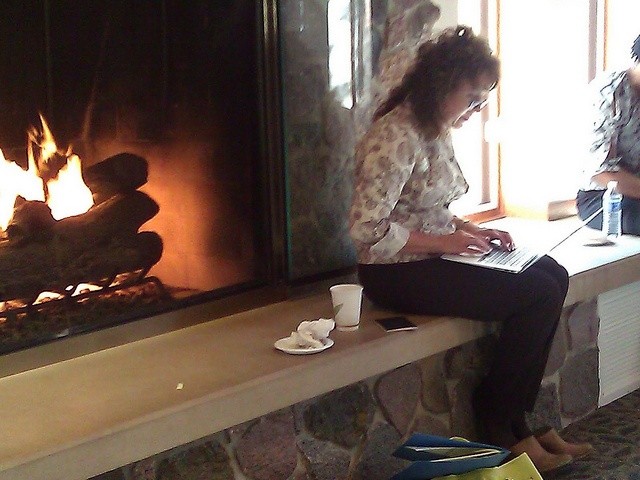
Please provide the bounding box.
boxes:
[603,181,623,242]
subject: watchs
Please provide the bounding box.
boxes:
[456,219,471,228]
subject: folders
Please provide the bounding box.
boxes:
[390,432,511,480]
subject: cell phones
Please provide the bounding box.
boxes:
[373,316,418,332]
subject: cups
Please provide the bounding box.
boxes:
[330,284,363,327]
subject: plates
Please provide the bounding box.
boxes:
[274,337,334,355]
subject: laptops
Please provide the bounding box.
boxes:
[441,206,603,275]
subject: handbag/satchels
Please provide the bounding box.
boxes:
[432,437,544,479]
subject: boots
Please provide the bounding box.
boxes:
[508,435,574,473]
[534,426,594,460]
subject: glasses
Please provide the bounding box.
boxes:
[465,95,488,112]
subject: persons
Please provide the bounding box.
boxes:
[346,23,594,476]
[576,35,640,236]
